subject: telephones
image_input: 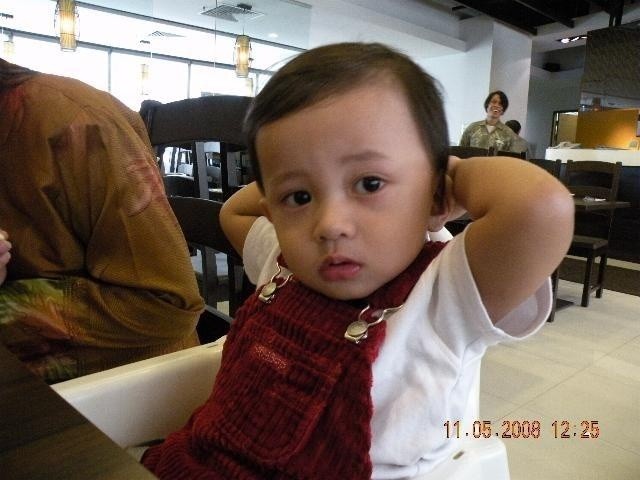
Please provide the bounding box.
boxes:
[558,142,582,149]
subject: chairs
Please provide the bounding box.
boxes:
[46,95,261,452]
[451,145,622,310]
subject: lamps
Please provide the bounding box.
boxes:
[231,3,254,77]
[51,1,85,53]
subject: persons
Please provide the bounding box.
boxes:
[505,118,534,161]
[138,37,576,479]
[1,54,209,383]
[458,90,516,156]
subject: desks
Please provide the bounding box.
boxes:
[572,194,630,214]
[0,346,159,480]
